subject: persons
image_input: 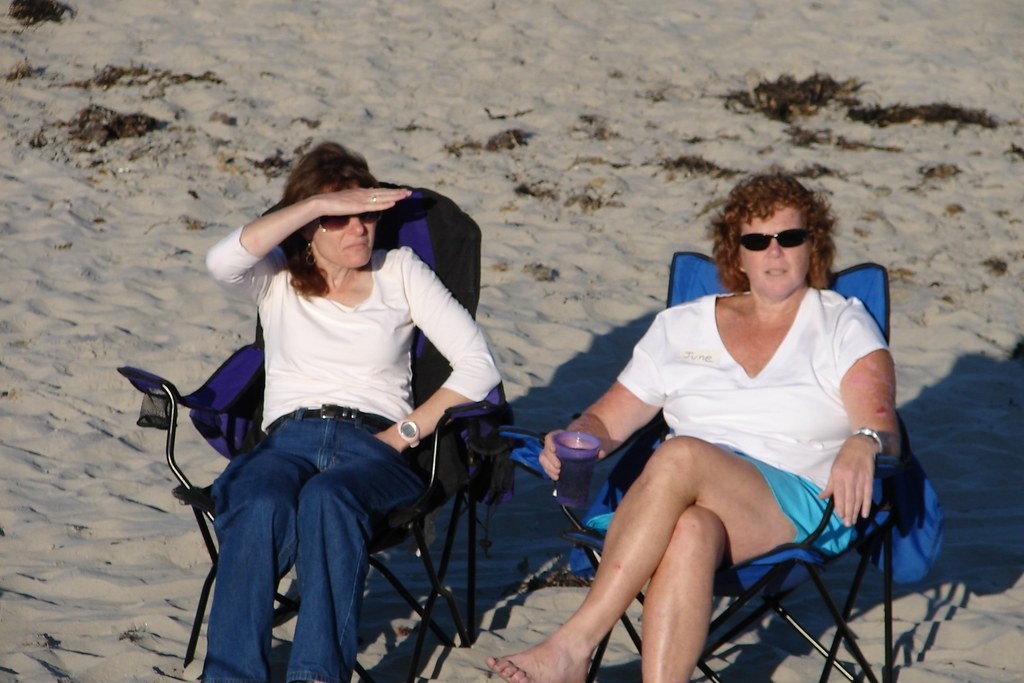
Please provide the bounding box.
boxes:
[484,174,900,683]
[208,142,501,682]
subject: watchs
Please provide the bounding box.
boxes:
[398,420,421,448]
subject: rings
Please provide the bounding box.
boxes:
[372,196,376,203]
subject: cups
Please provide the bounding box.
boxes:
[551,431,602,507]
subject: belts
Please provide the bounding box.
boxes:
[286,403,393,431]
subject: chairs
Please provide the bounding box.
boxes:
[122,183,947,683]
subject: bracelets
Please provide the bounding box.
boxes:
[852,428,883,453]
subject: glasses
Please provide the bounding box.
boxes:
[309,211,381,229]
[737,228,812,251]
